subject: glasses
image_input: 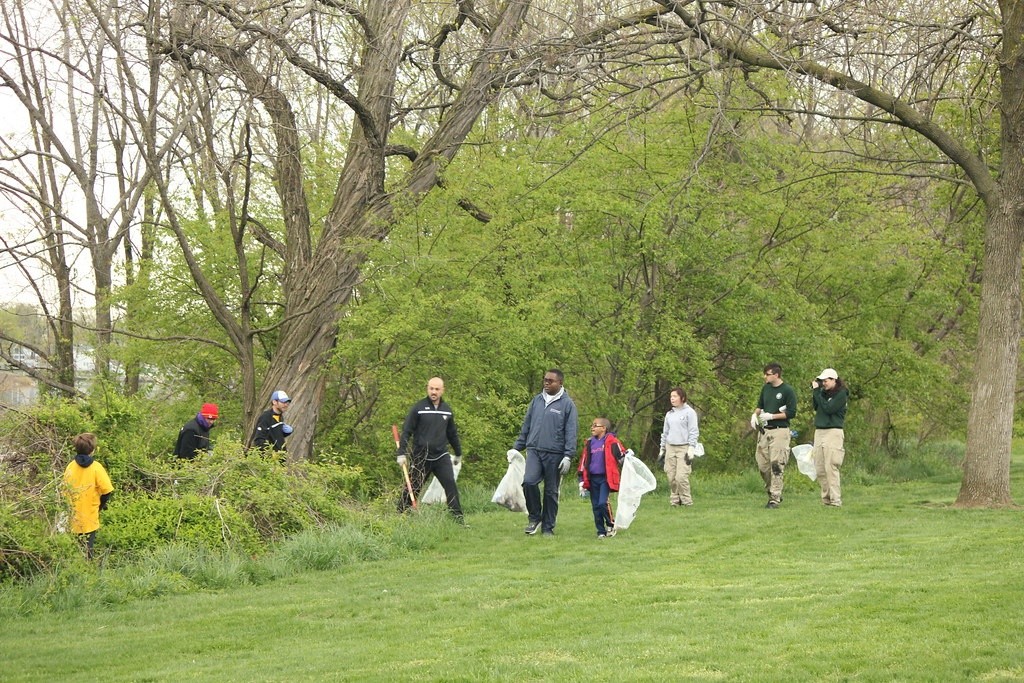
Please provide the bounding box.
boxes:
[765,372,773,375]
[543,378,558,384]
[591,423,604,428]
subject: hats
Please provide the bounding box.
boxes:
[817,369,838,380]
[201,403,218,418]
[271,391,291,403]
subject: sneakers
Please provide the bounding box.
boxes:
[597,533,605,540]
[526,519,541,534]
[606,526,617,538]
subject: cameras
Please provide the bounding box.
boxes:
[815,379,823,388]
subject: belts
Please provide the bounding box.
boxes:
[763,426,787,429]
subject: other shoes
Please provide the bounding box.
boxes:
[767,492,784,502]
[829,499,842,506]
[822,497,831,506]
[764,500,779,509]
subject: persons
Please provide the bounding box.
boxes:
[172,403,219,469]
[513,369,578,536]
[579,418,629,539]
[395,377,471,529]
[60,433,115,559]
[657,388,699,506]
[811,369,848,507]
[252,390,293,466]
[751,363,796,510]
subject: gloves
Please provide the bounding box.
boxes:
[759,413,772,422]
[453,454,461,465]
[684,446,693,466]
[625,448,634,456]
[656,448,667,461]
[751,413,759,430]
[579,482,588,497]
[397,454,407,466]
[557,456,572,475]
[283,423,294,434]
